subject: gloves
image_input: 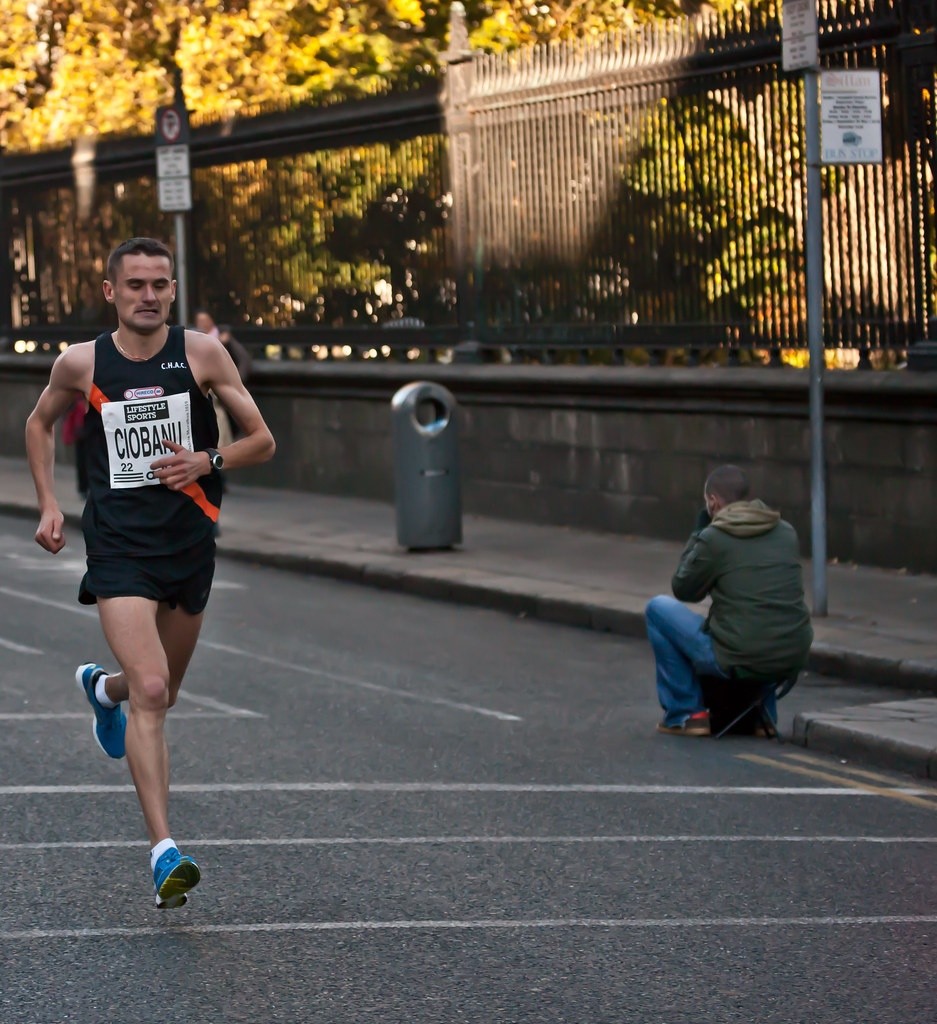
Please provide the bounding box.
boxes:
[695,509,711,529]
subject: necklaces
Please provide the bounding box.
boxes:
[116,324,169,361]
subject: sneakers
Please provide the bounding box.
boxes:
[656,707,711,734]
[153,847,201,908]
[74,663,127,759]
[754,721,776,738]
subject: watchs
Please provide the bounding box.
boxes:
[204,447,224,474]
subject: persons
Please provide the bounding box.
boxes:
[645,464,814,736]
[25,237,277,910]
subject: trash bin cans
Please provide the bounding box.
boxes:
[391,381,462,553]
